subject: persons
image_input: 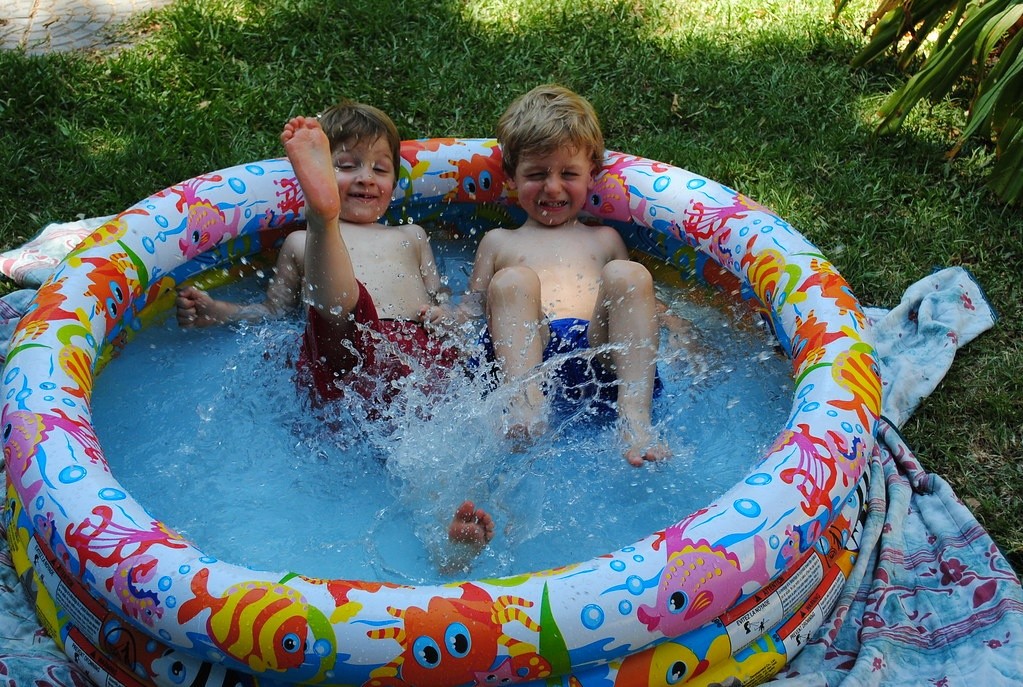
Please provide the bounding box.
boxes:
[176,102,494,549]
[459,84,664,467]
